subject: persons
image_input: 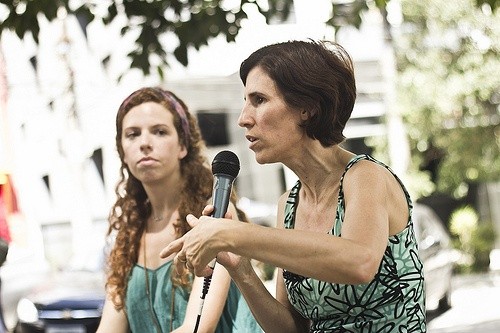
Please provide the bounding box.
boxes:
[0,179,10,332]
[92,86,244,333]
[160,40,427,333]
[216,224,275,332]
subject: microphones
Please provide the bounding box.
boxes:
[200,151,240,294]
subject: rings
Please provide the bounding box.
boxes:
[186,263,194,271]
[178,257,186,263]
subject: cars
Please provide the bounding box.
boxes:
[411,202,453,320]
[14,268,106,333]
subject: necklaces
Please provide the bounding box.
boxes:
[154,217,162,221]
[143,227,175,333]
